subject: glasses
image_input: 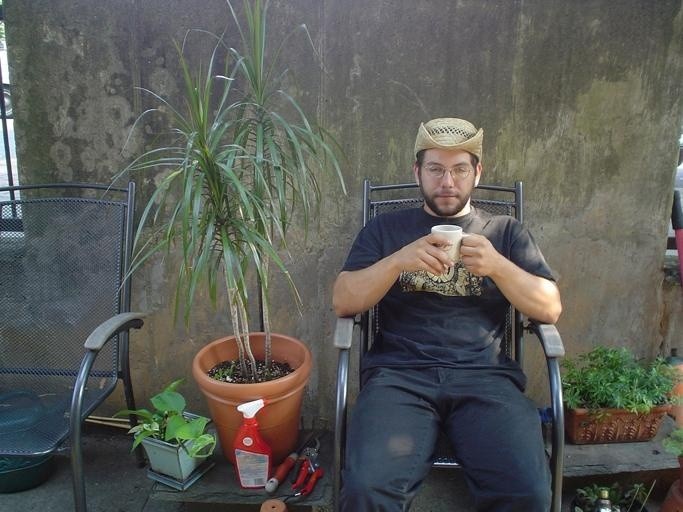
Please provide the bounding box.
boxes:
[422,165,472,179]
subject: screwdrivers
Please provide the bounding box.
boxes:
[265,429,315,492]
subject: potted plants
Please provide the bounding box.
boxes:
[560,345,683,445]
[571,483,648,512]
[105,1,351,468]
[112,378,218,493]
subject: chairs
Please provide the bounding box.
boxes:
[332,178,565,512]
[1,181,148,512]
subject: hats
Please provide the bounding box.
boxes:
[414,118,484,162]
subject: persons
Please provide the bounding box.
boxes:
[333,118,562,511]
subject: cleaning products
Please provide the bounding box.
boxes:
[235,398,272,489]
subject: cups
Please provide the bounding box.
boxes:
[432,225,469,266]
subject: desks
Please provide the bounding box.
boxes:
[148,428,335,512]
